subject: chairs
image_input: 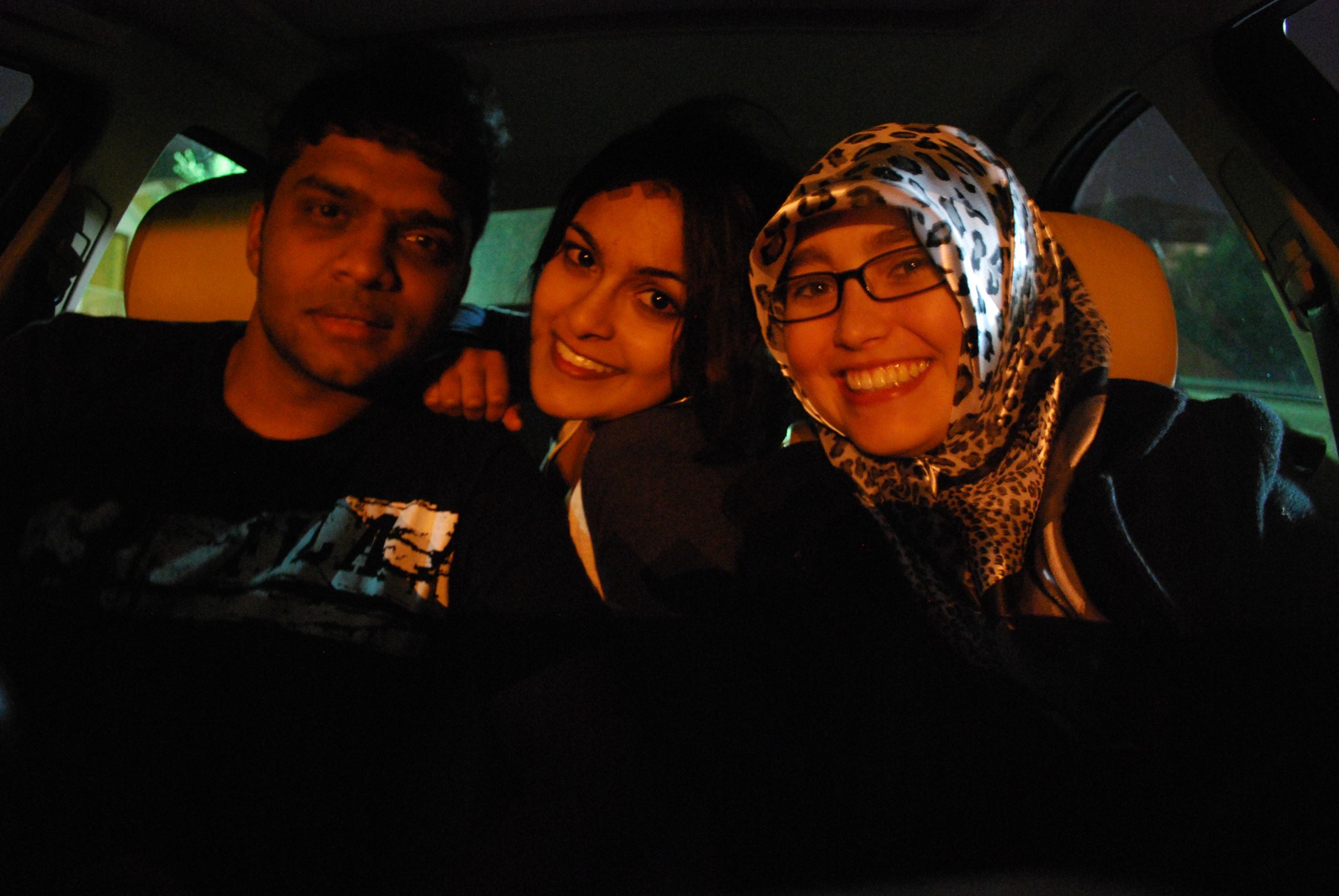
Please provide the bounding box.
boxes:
[126,175,261,321]
[1042,213,1175,384]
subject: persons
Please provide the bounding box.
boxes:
[747,116,1338,896]
[4,70,547,896]
[422,115,798,896]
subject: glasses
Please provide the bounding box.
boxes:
[771,248,948,323]
